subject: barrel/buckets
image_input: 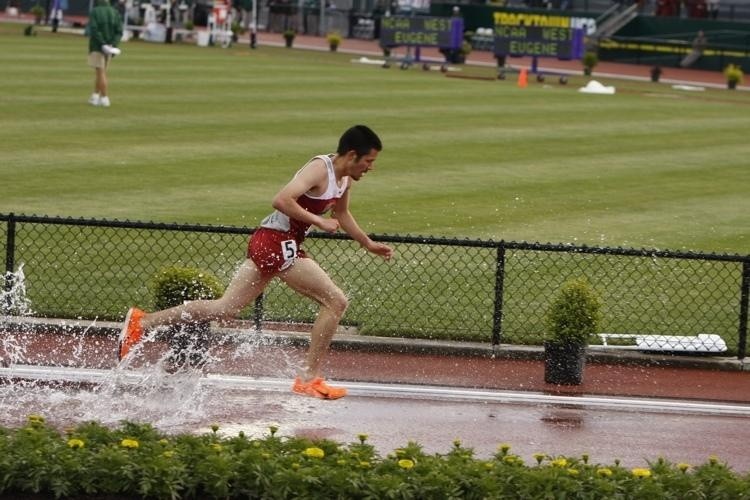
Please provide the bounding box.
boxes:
[197,31,211,46]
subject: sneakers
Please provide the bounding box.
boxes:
[88,93,110,106]
[292,376,347,401]
[116,308,146,362]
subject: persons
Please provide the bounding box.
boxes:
[87,0,123,107]
[49,5,63,32]
[116,125,393,402]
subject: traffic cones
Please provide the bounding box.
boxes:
[517,65,528,86]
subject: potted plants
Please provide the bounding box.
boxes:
[438,39,472,64]
[580,49,598,76]
[722,62,741,90]
[283,30,297,48]
[648,65,663,83]
[154,269,221,366]
[541,283,599,387]
[492,52,508,69]
[325,32,342,52]
[379,40,394,57]
